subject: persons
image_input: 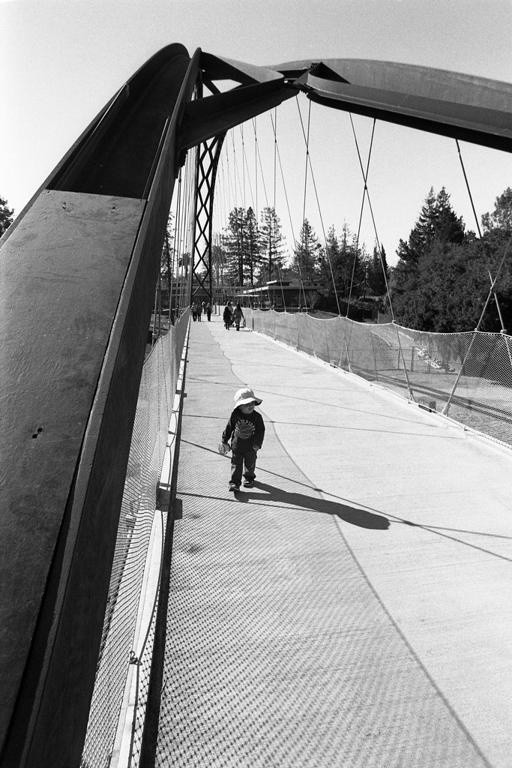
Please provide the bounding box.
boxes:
[220,386,265,491]
[189,298,266,332]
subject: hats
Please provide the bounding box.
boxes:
[233,389,261,409]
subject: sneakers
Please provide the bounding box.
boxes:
[243,480,255,486]
[229,486,239,492]
